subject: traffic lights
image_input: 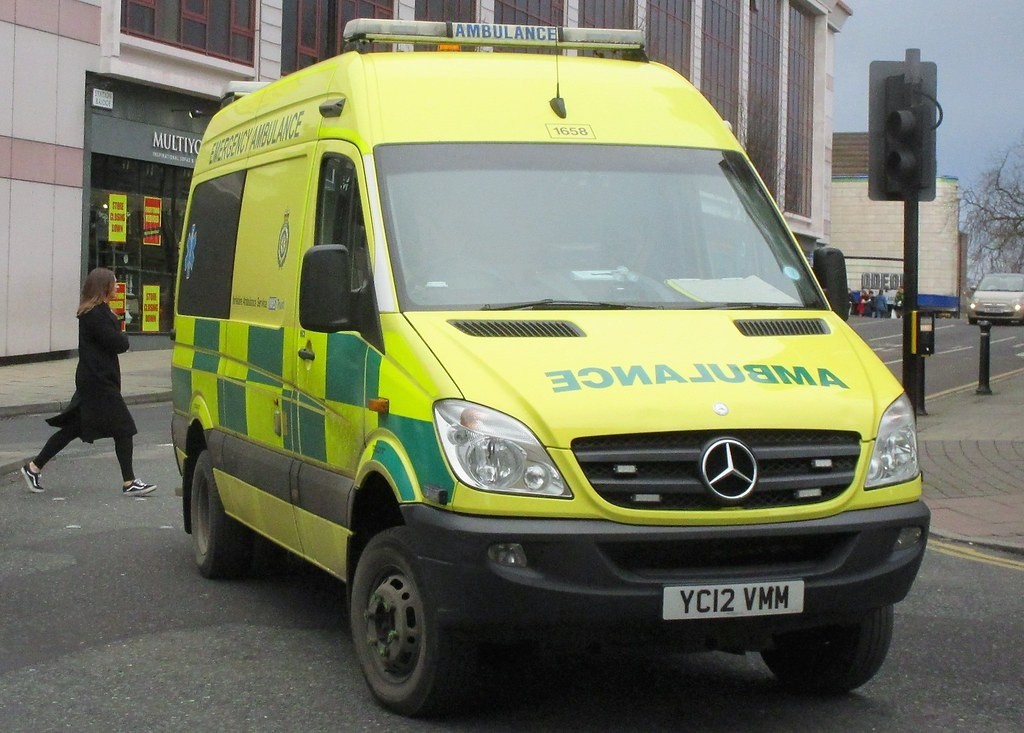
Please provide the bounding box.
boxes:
[870,61,938,203]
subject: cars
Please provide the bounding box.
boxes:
[967,274,1024,326]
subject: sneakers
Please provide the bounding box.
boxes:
[122,478,158,497]
[20,463,45,493]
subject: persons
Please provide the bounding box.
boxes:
[847,285,904,318]
[21,266,157,495]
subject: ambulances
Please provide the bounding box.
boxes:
[169,19,932,720]
